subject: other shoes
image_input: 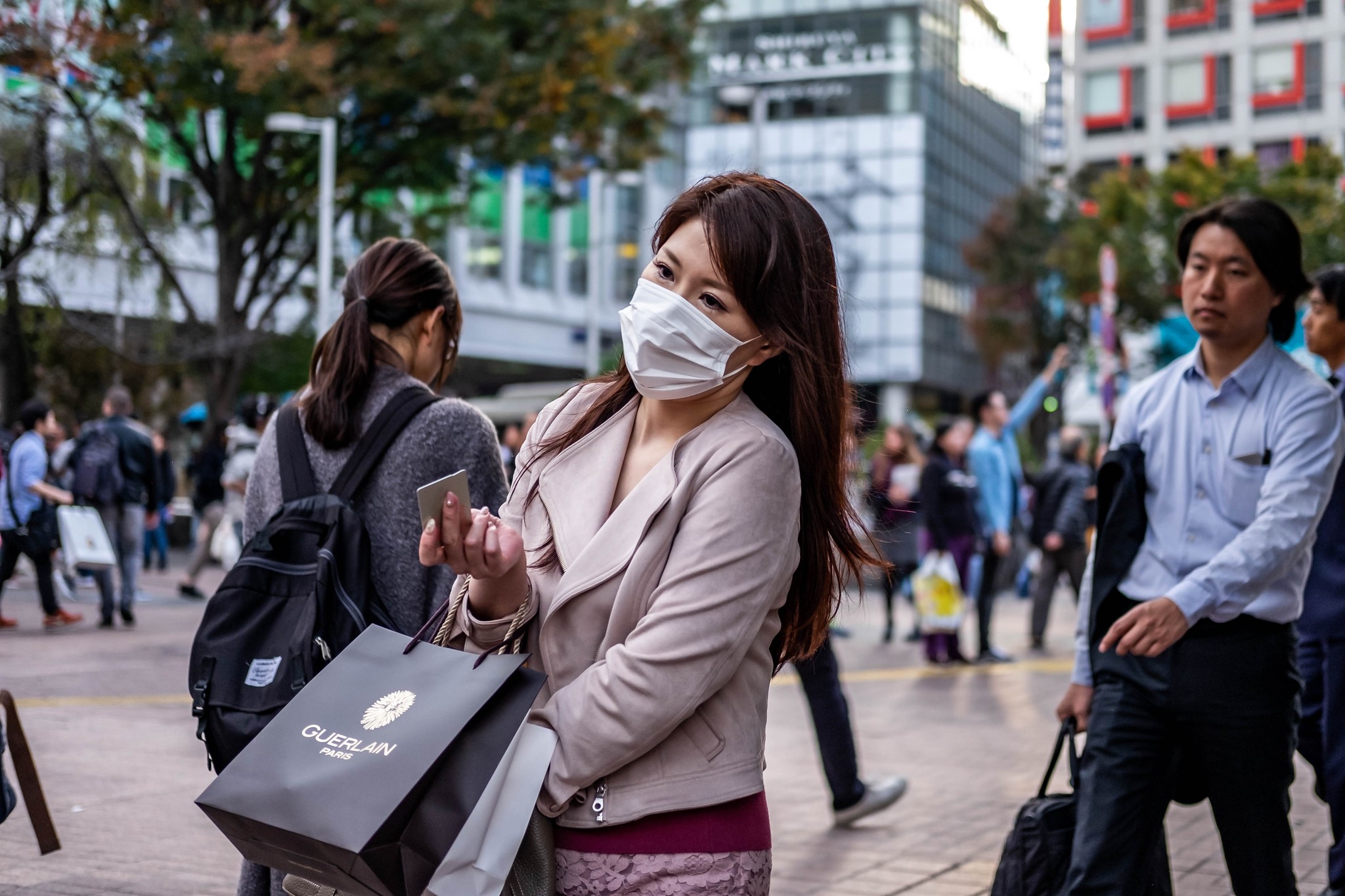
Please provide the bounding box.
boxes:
[178,584,204,600]
[833,776,912,829]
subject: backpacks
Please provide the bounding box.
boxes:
[72,424,126,503]
[188,387,442,774]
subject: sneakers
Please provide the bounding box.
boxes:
[54,569,79,602]
[44,609,86,631]
[0,616,19,632]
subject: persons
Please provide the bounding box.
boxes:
[864,337,1109,666]
[224,233,508,896]
[414,172,849,896]
[1053,199,1341,896]
[0,387,278,630]
[1297,270,1343,895]
[771,627,914,832]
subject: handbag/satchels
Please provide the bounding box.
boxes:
[194,599,550,896]
[912,549,968,636]
[208,513,242,572]
[991,716,1176,896]
[54,505,116,572]
[19,504,59,555]
[1012,548,1043,600]
[281,710,558,896]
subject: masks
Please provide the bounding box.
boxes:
[619,277,766,400]
